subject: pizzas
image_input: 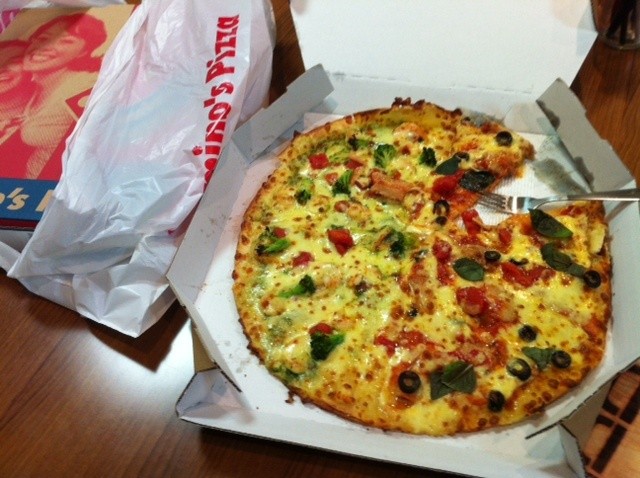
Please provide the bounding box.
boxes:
[230,97,614,437]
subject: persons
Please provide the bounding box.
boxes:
[18,13,107,180]
[0,38,42,179]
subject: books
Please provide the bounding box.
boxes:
[1,2,136,232]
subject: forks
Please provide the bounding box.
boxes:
[473,187,640,215]
